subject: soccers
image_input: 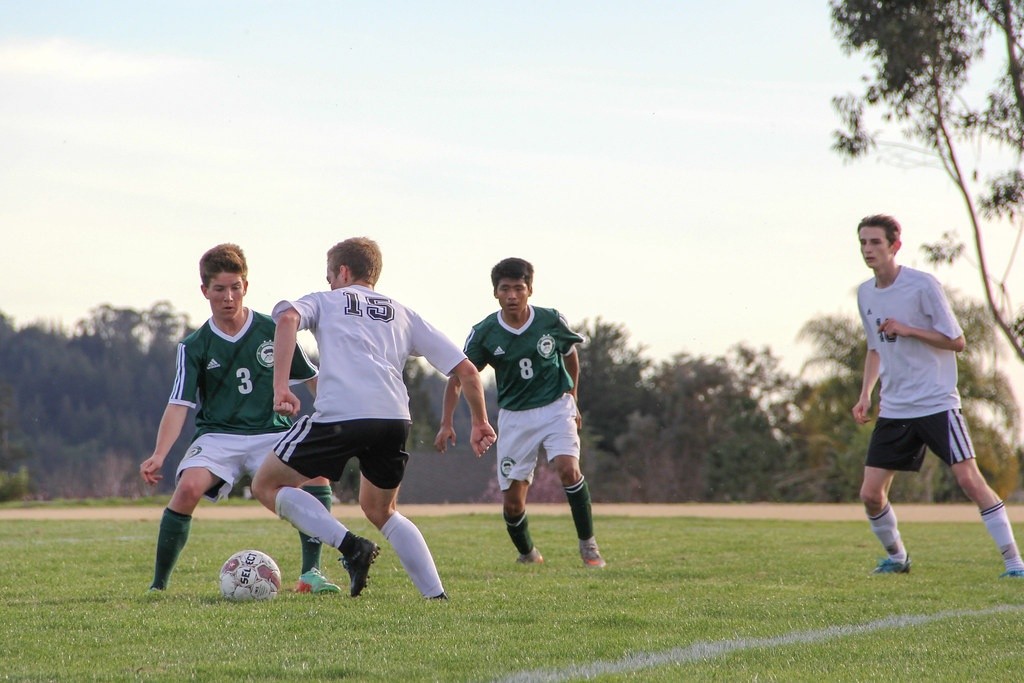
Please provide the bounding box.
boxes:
[218,550,282,602]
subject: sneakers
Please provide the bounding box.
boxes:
[433,592,449,599]
[296,569,341,594]
[1000,569,1024,577]
[515,546,543,565]
[579,538,606,569]
[874,551,913,577]
[339,536,380,598]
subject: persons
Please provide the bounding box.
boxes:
[432,257,607,569]
[251,235,498,599]
[137,242,341,595]
[852,214,1023,576]
